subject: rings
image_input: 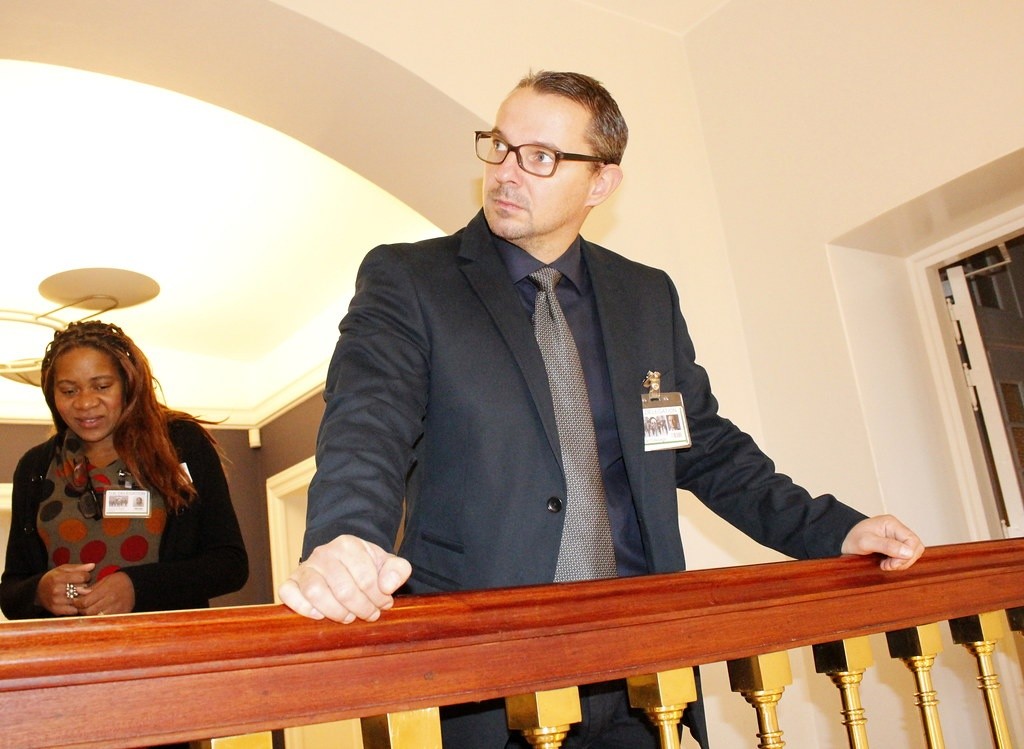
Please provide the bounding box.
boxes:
[65,584,79,599]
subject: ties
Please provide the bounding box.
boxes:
[531,267,617,583]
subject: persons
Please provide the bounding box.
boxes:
[279,71,924,749]
[0,321,250,620]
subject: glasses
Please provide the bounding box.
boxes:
[72,456,102,521]
[475,131,619,177]
